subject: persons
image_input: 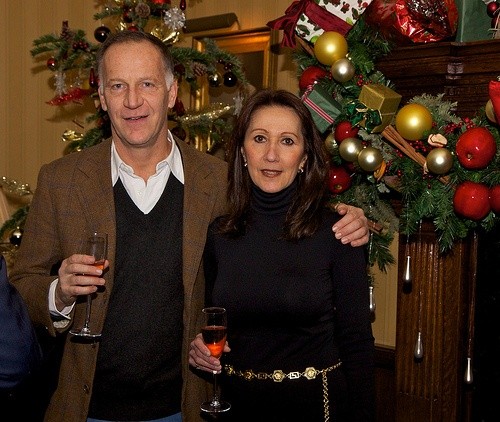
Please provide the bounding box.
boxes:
[188,89,375,422]
[8,30,370,422]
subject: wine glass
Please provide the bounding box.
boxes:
[200,307,231,413]
[69,231,108,337]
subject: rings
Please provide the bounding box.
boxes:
[195,364,199,369]
